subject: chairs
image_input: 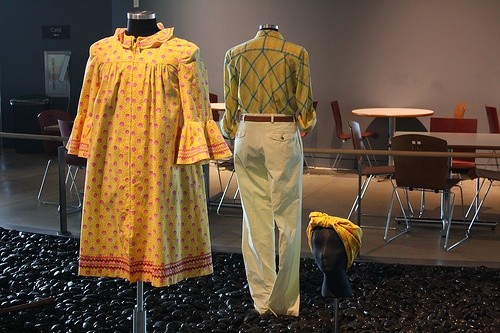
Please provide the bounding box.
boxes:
[35,93,500,252]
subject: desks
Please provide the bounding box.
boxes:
[209,103,241,122]
[394,131,500,238]
[351,108,435,179]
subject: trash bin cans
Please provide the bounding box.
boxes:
[10,96,48,153]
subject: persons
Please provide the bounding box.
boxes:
[220,24,317,316]
[64,10,234,288]
[306,211,363,297]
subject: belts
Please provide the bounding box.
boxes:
[240,115,296,122]
